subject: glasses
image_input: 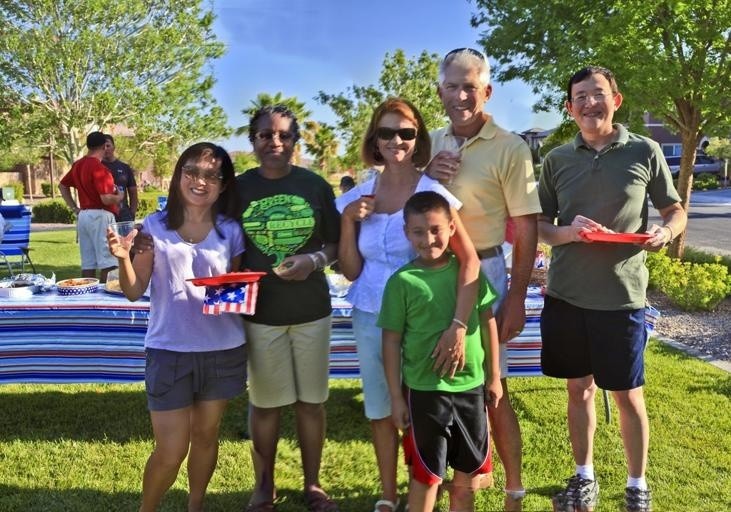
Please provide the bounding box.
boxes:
[570,91,612,100]
[444,47,485,62]
[375,126,417,141]
[179,165,222,183]
[253,131,291,140]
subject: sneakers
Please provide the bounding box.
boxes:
[552,475,601,511]
[624,482,654,512]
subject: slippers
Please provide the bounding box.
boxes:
[252,499,274,512]
[307,495,338,512]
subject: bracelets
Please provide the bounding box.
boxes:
[453,317,469,330]
[307,250,317,272]
[315,251,329,266]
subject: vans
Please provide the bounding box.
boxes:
[665,155,721,179]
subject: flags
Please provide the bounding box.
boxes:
[202,282,259,316]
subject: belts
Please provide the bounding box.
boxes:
[478,245,504,260]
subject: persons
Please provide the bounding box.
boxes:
[414,49,543,512]
[106,143,246,512]
[58,132,125,284]
[375,191,504,512]
[334,99,482,512]
[340,177,355,193]
[129,105,339,511]
[101,135,138,221]
[536,68,687,512]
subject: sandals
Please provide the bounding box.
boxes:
[373,496,402,512]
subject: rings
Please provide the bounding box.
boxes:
[514,330,520,333]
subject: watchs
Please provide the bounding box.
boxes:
[665,224,674,245]
[315,254,324,272]
[72,206,77,210]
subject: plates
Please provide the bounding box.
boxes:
[185,272,268,287]
[103,285,126,296]
[0,281,40,297]
[55,278,99,295]
[579,230,656,243]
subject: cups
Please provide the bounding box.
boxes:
[357,168,380,199]
[442,136,468,187]
[106,221,134,247]
